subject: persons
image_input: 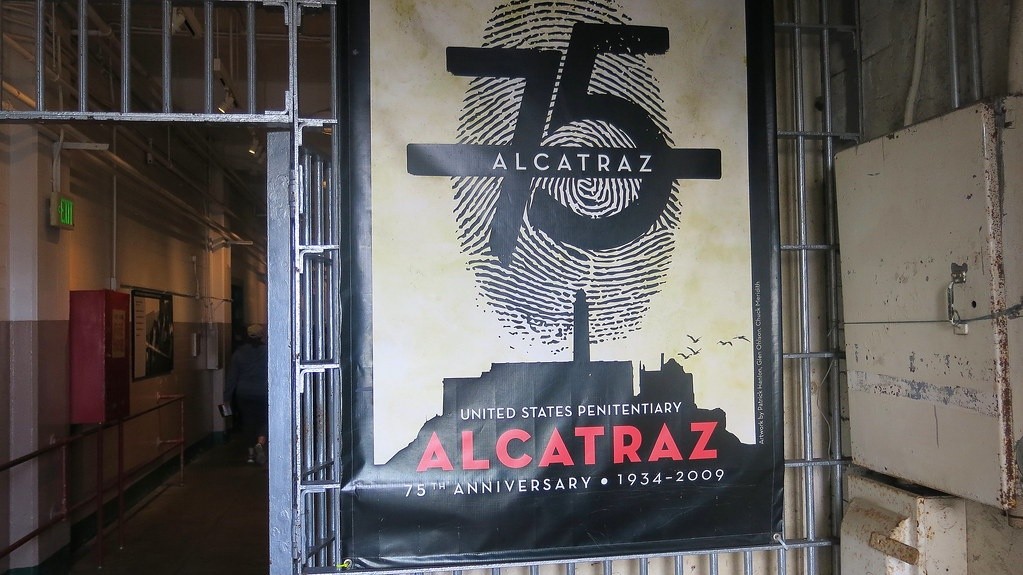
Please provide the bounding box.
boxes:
[224,324,268,466]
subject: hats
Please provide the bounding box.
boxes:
[247,324,264,338]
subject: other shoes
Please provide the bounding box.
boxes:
[254,442,266,465]
[247,455,255,463]
